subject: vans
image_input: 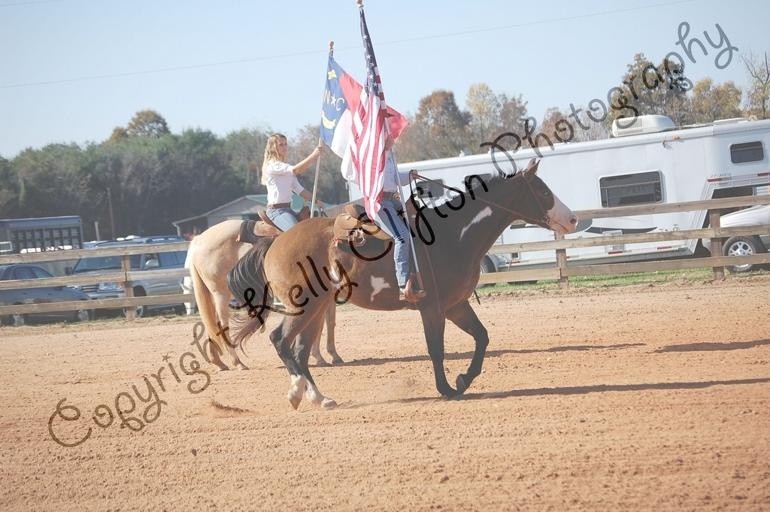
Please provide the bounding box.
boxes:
[348,113,769,289]
[70,237,196,320]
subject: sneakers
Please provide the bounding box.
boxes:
[399,277,426,302]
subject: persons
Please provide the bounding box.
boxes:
[341,136,427,302]
[261,133,325,233]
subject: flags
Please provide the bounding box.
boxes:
[319,50,409,160]
[350,8,388,224]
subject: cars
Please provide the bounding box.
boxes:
[0,265,100,326]
[702,203,770,272]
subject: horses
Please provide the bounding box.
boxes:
[185,192,404,371]
[226,157,578,410]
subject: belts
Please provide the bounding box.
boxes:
[268,203,291,209]
[381,190,401,202]
